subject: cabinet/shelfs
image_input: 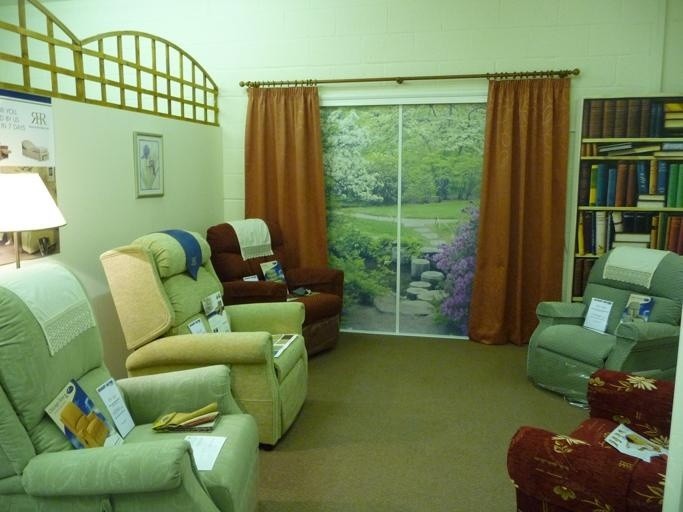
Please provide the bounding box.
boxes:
[569,93,683,302]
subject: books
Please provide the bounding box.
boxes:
[571,99,683,296]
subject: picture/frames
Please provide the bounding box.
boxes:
[133,132,165,198]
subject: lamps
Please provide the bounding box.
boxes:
[0,172,68,268]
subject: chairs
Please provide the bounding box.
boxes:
[527,246,682,404]
[507,368,674,512]
